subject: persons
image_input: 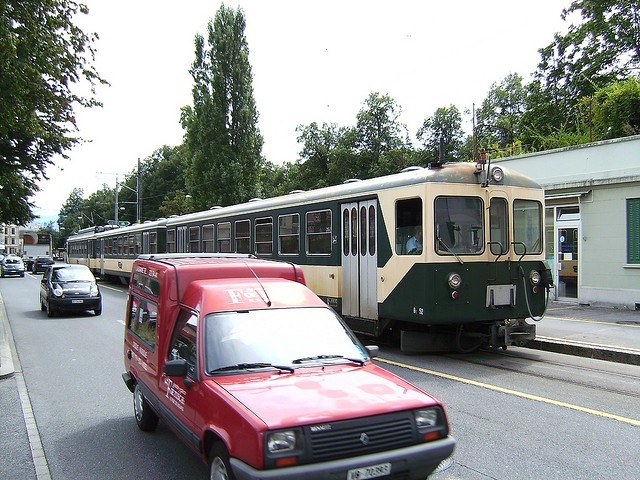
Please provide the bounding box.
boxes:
[406,226,422,253]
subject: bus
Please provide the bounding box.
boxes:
[62,147,555,355]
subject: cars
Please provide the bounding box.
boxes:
[0,256,26,278]
[21,256,35,271]
[31,255,56,275]
[39,264,102,318]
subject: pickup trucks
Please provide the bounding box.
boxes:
[121,252,456,480]
